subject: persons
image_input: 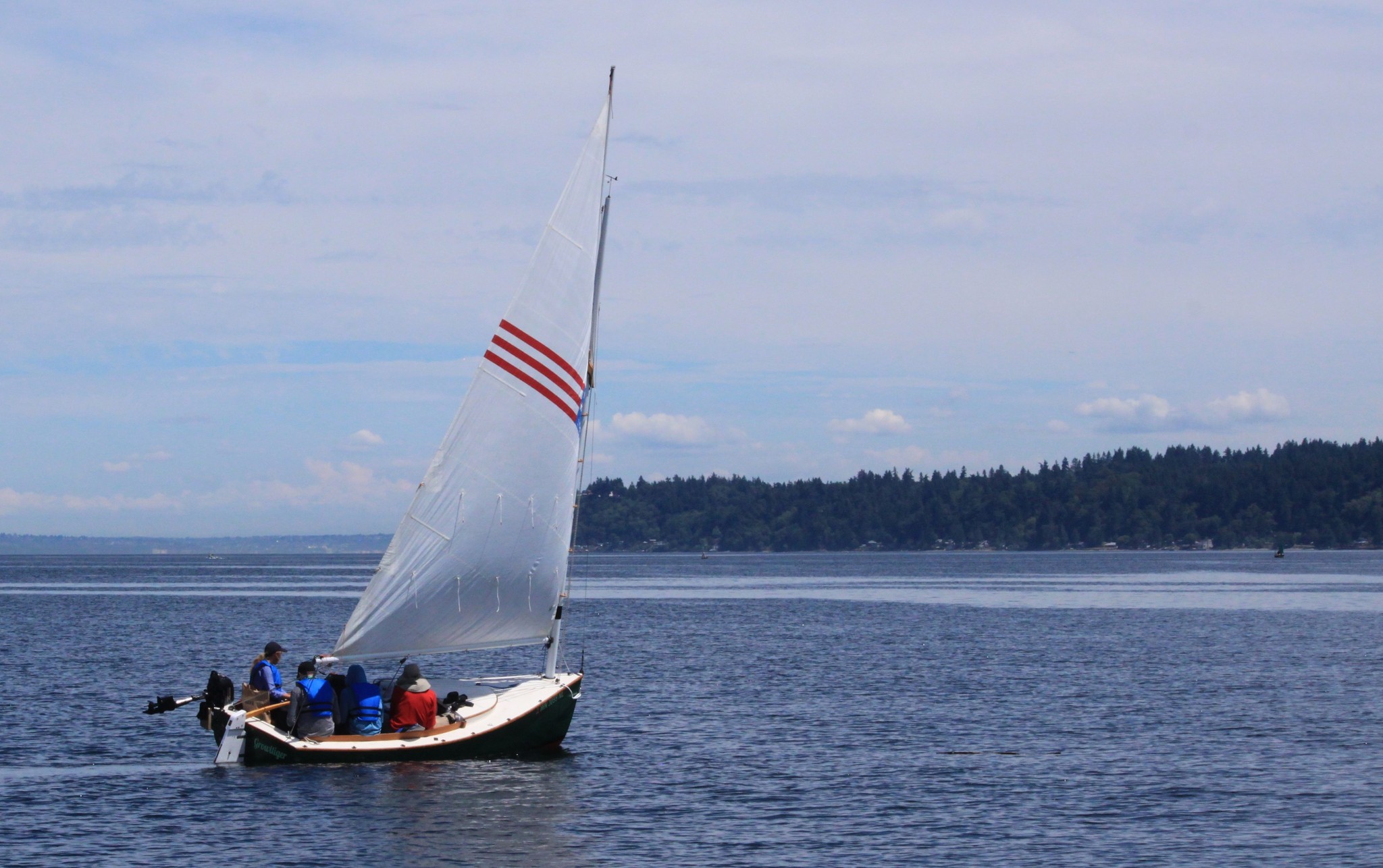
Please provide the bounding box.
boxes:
[385,663,437,732]
[337,665,384,736]
[249,641,291,732]
[286,661,341,739]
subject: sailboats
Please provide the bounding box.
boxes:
[200,67,621,765]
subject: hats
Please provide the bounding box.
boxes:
[296,661,316,680]
[265,642,288,657]
[396,663,431,692]
